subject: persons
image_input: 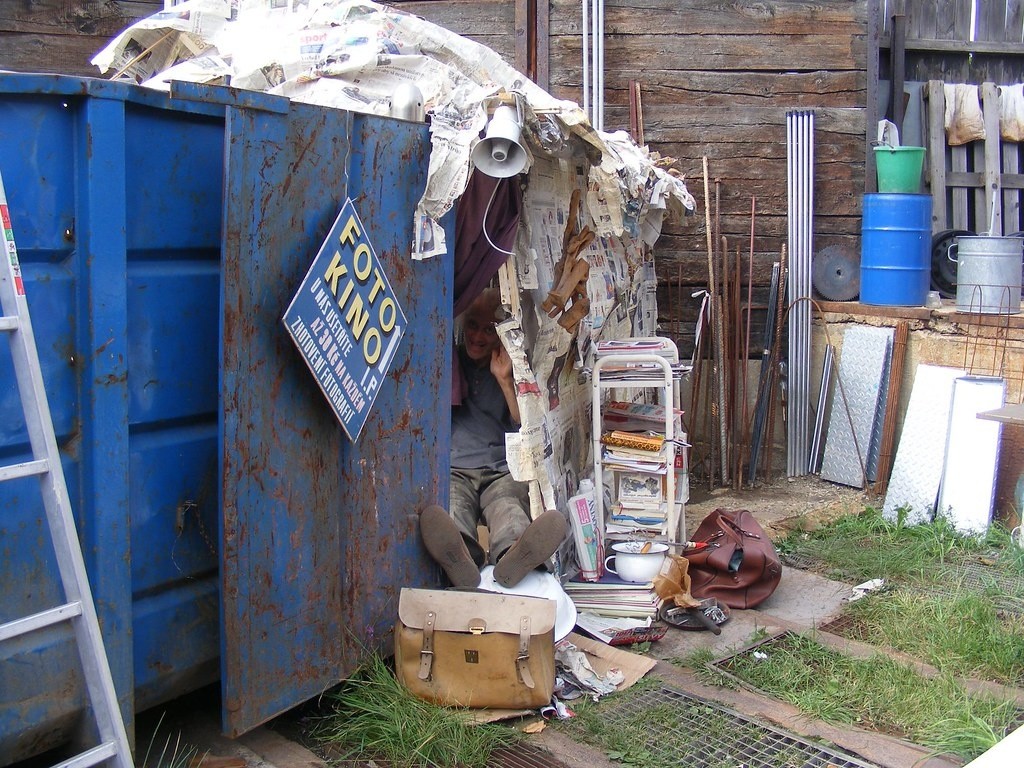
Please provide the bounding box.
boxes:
[420,287,567,591]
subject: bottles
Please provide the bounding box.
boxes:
[926,289,942,307]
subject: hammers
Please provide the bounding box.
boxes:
[666,595,722,636]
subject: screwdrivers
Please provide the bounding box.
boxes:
[660,538,711,549]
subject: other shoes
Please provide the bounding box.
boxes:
[493,510,567,588]
[419,505,481,587]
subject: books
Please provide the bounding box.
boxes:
[563,340,693,622]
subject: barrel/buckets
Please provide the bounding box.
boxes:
[859,193,934,307]
[948,236,1024,314]
[870,140,927,194]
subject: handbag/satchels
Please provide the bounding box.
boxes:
[681,508,783,609]
[393,586,556,710]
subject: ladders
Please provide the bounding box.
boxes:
[0,161,147,768]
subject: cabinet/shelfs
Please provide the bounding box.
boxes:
[590,356,688,563]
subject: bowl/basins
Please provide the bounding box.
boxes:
[603,543,669,583]
